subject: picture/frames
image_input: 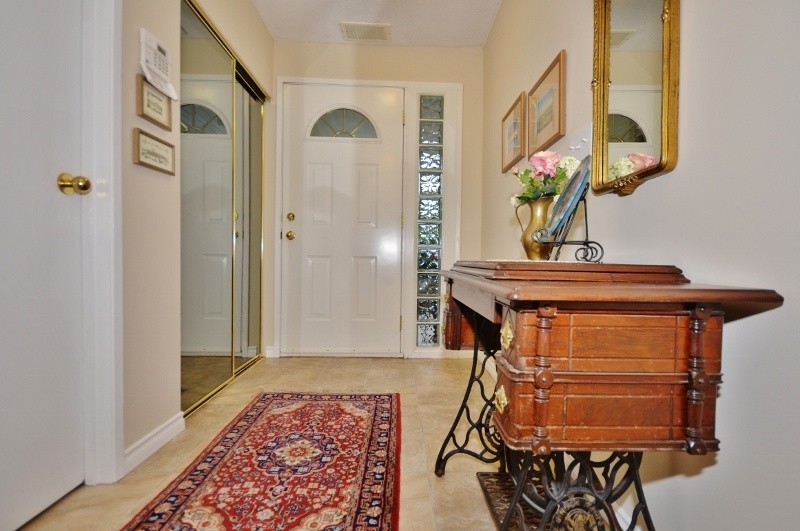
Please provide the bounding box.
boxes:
[134,73,177,133]
[500,92,528,174]
[527,49,566,159]
[133,127,176,178]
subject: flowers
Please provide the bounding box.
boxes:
[510,148,580,206]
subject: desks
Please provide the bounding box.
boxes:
[444,257,783,458]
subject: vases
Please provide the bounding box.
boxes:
[516,196,554,260]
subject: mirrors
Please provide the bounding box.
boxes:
[592,1,680,200]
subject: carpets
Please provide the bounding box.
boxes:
[119,388,401,531]
